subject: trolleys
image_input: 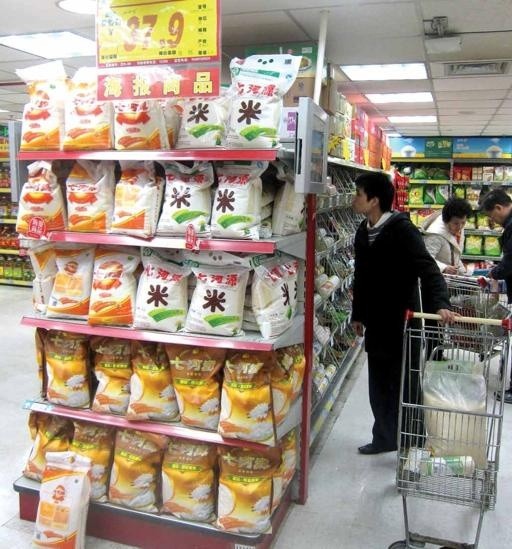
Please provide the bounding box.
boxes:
[387,273,511,548]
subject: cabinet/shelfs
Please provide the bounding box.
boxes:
[0,120,40,286]
[12,148,512,549]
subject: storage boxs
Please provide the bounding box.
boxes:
[244,42,392,171]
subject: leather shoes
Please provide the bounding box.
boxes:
[358,443,398,454]
[494,389,512,404]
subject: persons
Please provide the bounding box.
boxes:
[349,171,465,456]
[478,187,511,403]
[419,195,473,360]
